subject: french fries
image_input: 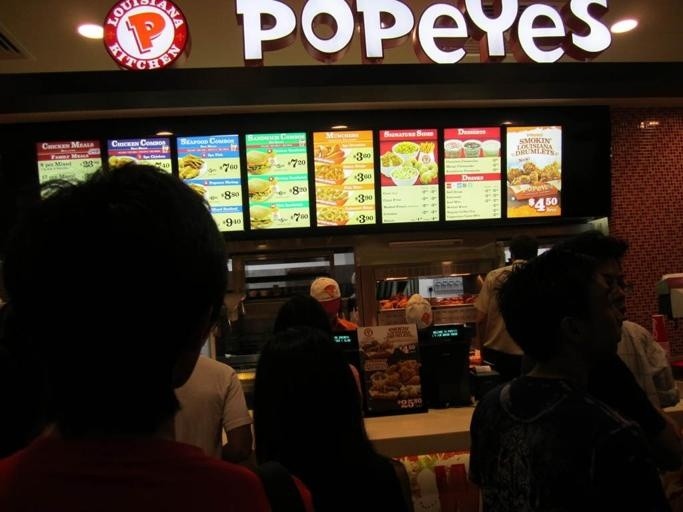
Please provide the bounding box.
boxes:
[420,140,435,154]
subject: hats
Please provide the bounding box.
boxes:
[406,293,433,331]
[310,276,341,316]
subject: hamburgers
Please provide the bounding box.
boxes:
[246,152,271,175]
[248,179,273,202]
[250,206,273,227]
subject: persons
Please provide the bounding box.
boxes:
[549,231,682,473]
[173,356,254,463]
[311,277,359,328]
[406,293,468,409]
[469,229,680,512]
[616,275,674,410]
[275,298,363,408]
[1,162,312,512]
[474,234,539,376]
[243,326,415,512]
